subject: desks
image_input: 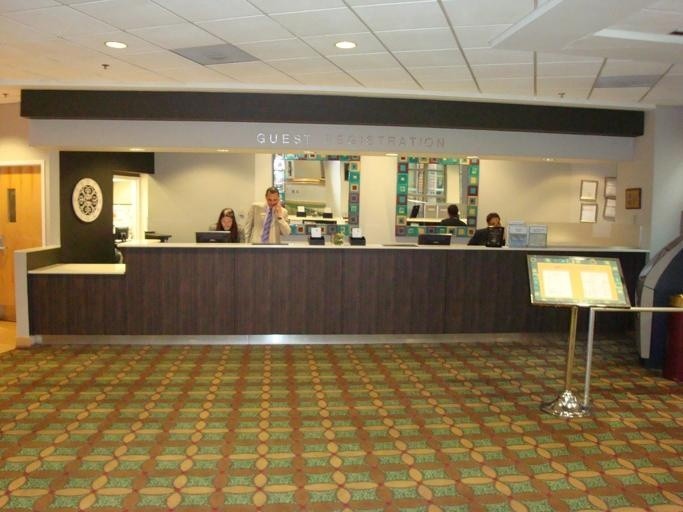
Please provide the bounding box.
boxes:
[113,233,172,243]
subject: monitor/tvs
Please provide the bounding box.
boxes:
[418,234,451,245]
[196,231,232,242]
[115,226,129,241]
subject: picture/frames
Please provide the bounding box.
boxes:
[625,188,641,209]
[603,197,617,220]
[604,177,617,198]
[580,181,598,201]
[580,204,597,222]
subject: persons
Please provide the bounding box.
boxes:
[208,208,244,243]
[467,213,505,246]
[439,205,467,226]
[244,187,291,244]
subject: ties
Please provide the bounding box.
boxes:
[261,209,272,242]
